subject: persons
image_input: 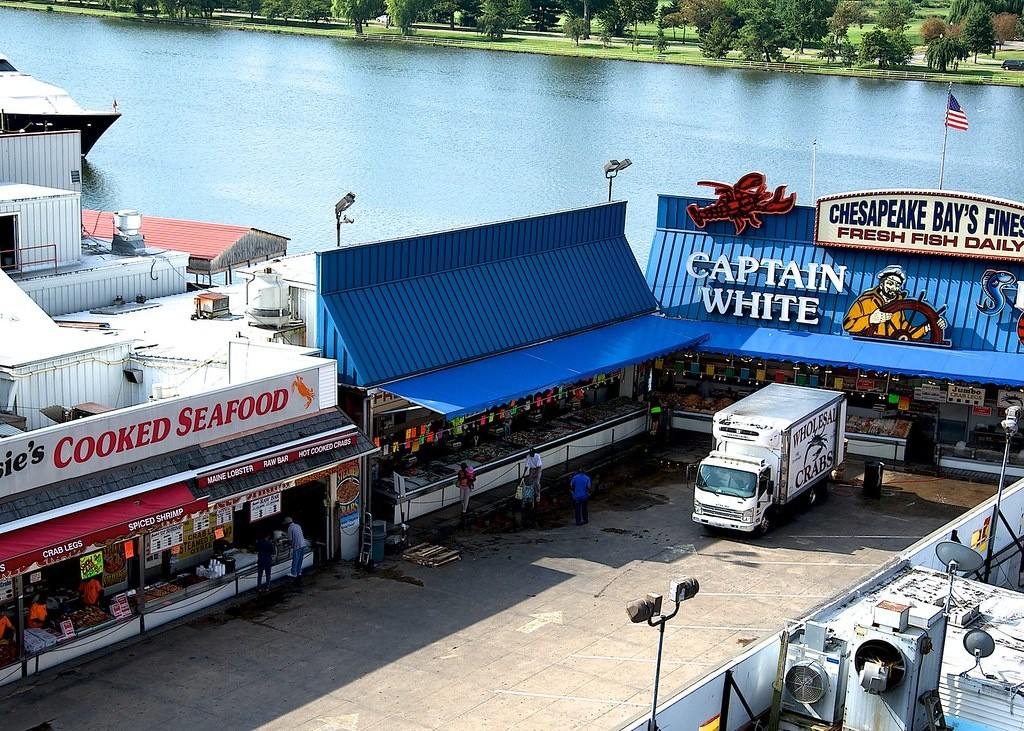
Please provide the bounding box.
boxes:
[0,605,17,653]
[213,532,230,557]
[274,527,288,540]
[79,578,105,608]
[570,466,594,527]
[27,593,54,629]
[254,530,276,594]
[457,462,475,516]
[276,517,305,579]
[699,376,715,398]
[520,449,542,515]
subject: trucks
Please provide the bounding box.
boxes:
[685,381,848,539]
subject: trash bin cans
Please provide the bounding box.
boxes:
[863,460,884,500]
[364,521,387,563]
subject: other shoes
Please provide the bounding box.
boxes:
[298,572,301,575]
[266,587,269,591]
[535,496,540,502]
[650,430,656,435]
[286,572,297,577]
[258,587,262,592]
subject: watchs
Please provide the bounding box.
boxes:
[569,489,572,492]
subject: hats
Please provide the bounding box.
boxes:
[465,466,474,473]
[283,517,292,524]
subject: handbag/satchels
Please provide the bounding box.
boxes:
[456,480,460,487]
[515,478,525,499]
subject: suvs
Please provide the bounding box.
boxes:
[1000,59,1024,70]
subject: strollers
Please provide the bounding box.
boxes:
[521,476,541,509]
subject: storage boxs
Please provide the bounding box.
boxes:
[63,629,76,638]
[60,620,73,632]
[110,602,121,615]
[114,612,124,621]
[121,605,132,618]
[13,627,56,653]
[113,593,129,608]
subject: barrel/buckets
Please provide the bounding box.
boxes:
[152,383,162,399]
[863,460,885,500]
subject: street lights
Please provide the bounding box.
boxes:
[604,158,632,203]
[985,404,1024,584]
[625,575,700,731]
[334,190,356,246]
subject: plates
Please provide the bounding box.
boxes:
[336,477,359,505]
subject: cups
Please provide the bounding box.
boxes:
[208,559,225,577]
